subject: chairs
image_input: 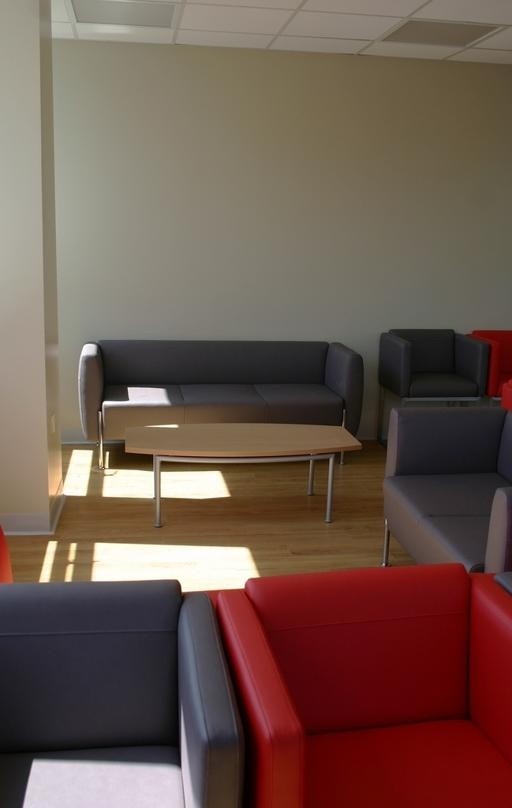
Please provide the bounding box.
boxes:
[469,331,511,410]
[378,328,492,440]
[0,526,12,585]
[0,581,245,806]
[219,563,511,808]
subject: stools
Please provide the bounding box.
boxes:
[494,572,511,595]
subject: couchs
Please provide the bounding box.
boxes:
[382,407,511,572]
[77,338,365,469]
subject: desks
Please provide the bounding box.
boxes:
[125,423,361,527]
